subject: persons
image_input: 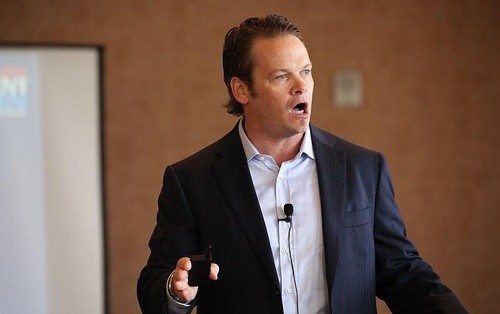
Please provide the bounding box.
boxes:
[133,13,468,314]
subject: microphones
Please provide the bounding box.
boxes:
[284,204,293,222]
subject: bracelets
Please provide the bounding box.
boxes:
[169,273,185,303]
